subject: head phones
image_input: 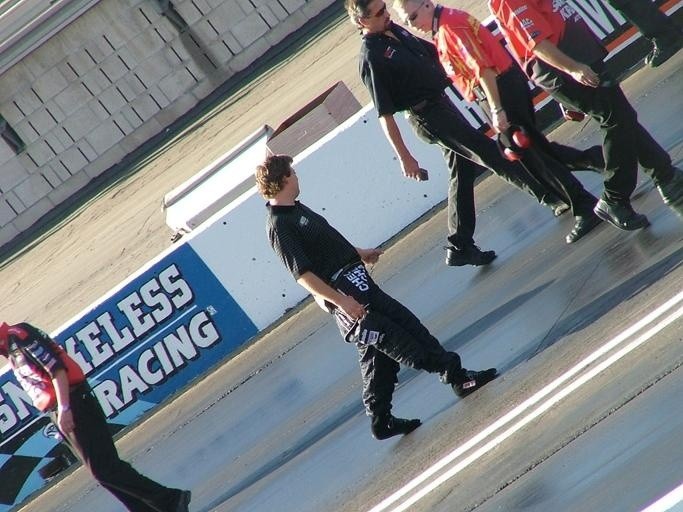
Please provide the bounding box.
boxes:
[559,103,585,122]
[497,123,534,162]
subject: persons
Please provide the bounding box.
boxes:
[1,319,192,512]
[342,0,570,269]
[392,0,604,246]
[252,153,497,442]
[605,0,683,71]
[486,0,682,232]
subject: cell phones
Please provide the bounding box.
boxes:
[419,168,428,180]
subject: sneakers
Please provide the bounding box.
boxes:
[548,200,569,218]
[656,167,683,204]
[451,368,496,398]
[644,35,683,68]
[371,415,420,440]
[586,145,605,174]
[592,197,648,231]
[564,215,603,244]
[443,245,496,266]
[176,490,191,511]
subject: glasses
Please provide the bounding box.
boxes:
[402,2,424,25]
[367,2,386,17]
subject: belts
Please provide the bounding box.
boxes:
[402,98,431,120]
